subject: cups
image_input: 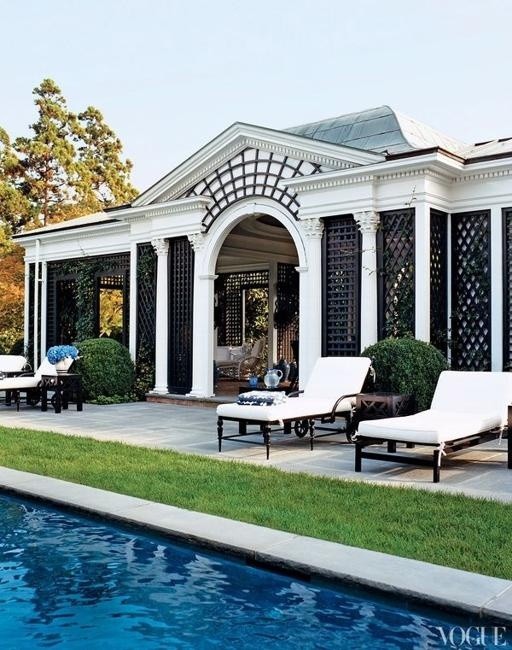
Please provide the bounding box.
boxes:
[249,375,258,386]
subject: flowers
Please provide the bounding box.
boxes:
[46,344,78,365]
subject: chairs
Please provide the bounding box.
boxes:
[0,355,77,411]
[215,357,511,485]
[215,339,265,381]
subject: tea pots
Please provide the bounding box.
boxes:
[263,368,284,390]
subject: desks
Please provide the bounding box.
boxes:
[40,375,83,415]
